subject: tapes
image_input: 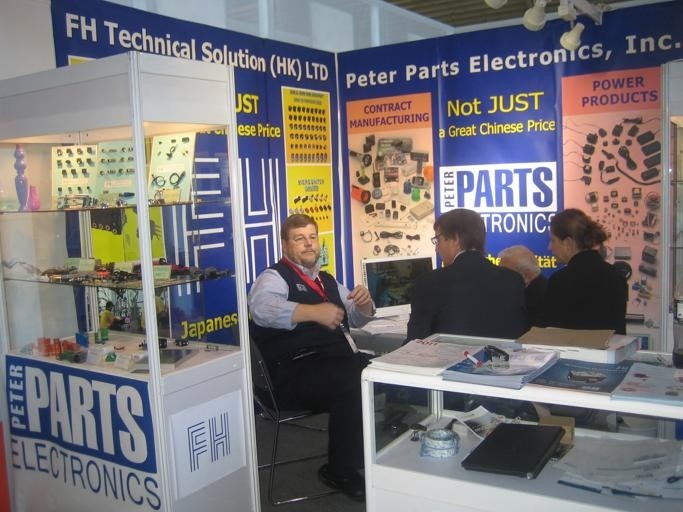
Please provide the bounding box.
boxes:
[423,429,459,458]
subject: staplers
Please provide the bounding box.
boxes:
[484,345,509,362]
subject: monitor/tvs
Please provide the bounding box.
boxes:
[362,256,434,322]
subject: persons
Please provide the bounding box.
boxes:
[387,208,551,420]
[100,301,118,330]
[494,244,547,321]
[529,207,629,430]
[137,286,165,331]
[246,213,387,502]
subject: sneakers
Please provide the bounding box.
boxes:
[318,462,365,502]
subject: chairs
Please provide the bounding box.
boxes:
[249,334,343,506]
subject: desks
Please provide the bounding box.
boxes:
[349,304,412,353]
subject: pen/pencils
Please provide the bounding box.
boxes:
[464,351,483,367]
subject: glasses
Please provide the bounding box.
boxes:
[430,233,442,245]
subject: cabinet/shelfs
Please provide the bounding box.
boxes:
[0,50,260,512]
[361,332,682,512]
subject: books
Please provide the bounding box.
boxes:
[460,422,566,481]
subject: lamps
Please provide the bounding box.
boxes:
[484,0,604,51]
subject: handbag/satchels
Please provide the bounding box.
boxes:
[275,338,357,372]
